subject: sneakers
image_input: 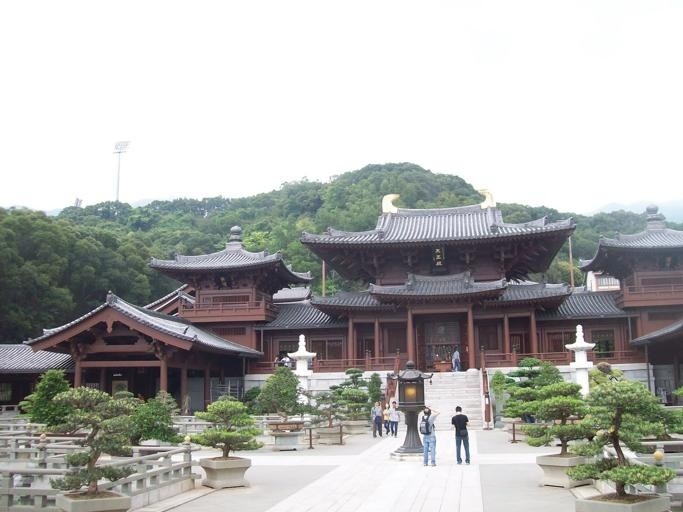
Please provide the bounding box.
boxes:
[385,429,397,438]
[372,434,382,438]
[456,459,470,465]
[423,464,436,467]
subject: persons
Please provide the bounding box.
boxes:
[449,348,461,371]
[419,405,440,467]
[279,352,291,367]
[273,353,280,367]
[370,400,383,437]
[450,406,471,464]
[382,402,390,435]
[386,400,402,436]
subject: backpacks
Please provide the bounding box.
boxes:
[419,415,430,435]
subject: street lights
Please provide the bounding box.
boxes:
[113,139,130,201]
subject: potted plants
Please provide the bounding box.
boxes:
[313,387,372,443]
[252,366,310,452]
[191,393,265,490]
[521,378,683,512]
[44,384,186,512]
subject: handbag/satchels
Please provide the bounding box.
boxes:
[375,416,382,424]
[459,429,467,436]
